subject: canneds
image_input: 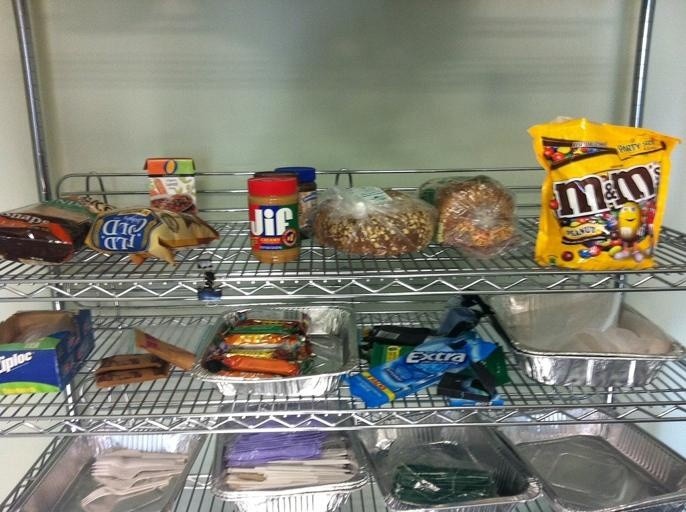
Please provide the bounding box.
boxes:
[248,176,301,263]
[275,166,317,240]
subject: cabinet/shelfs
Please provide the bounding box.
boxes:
[0,166,686,438]
